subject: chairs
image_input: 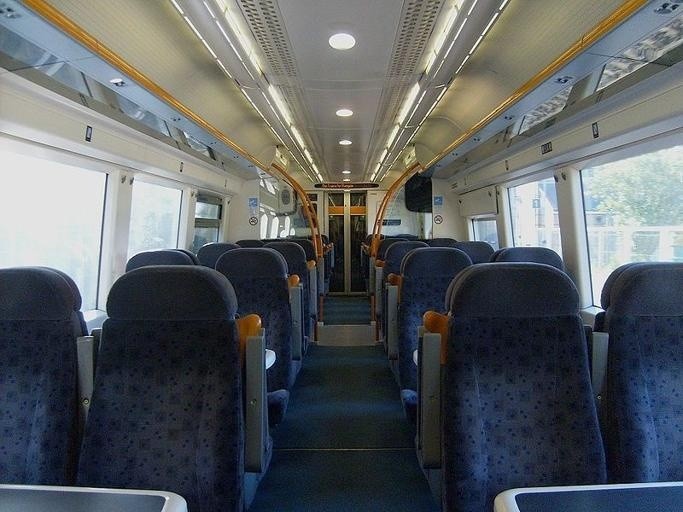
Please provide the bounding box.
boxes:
[0,235,332,512]
[362,232,683,512]
